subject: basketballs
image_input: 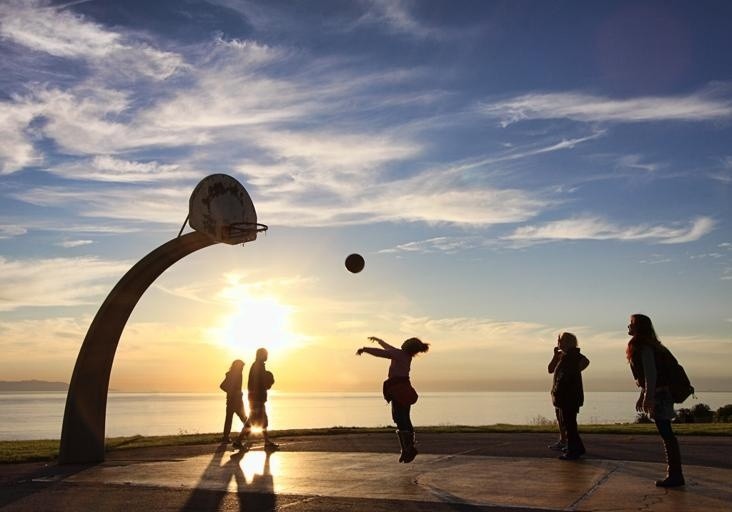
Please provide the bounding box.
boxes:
[345,254,364,273]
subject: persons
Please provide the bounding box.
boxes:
[220,359,253,442]
[231,345,281,452]
[355,332,432,463]
[624,311,697,491]
[546,330,589,461]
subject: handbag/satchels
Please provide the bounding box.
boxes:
[220,372,232,392]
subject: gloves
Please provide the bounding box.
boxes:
[635,391,644,412]
[642,392,655,418]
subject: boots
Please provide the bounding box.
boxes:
[396,430,418,463]
[655,437,684,487]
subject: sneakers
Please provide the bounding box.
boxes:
[548,435,586,460]
[222,434,280,449]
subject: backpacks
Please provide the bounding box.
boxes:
[658,343,694,404]
